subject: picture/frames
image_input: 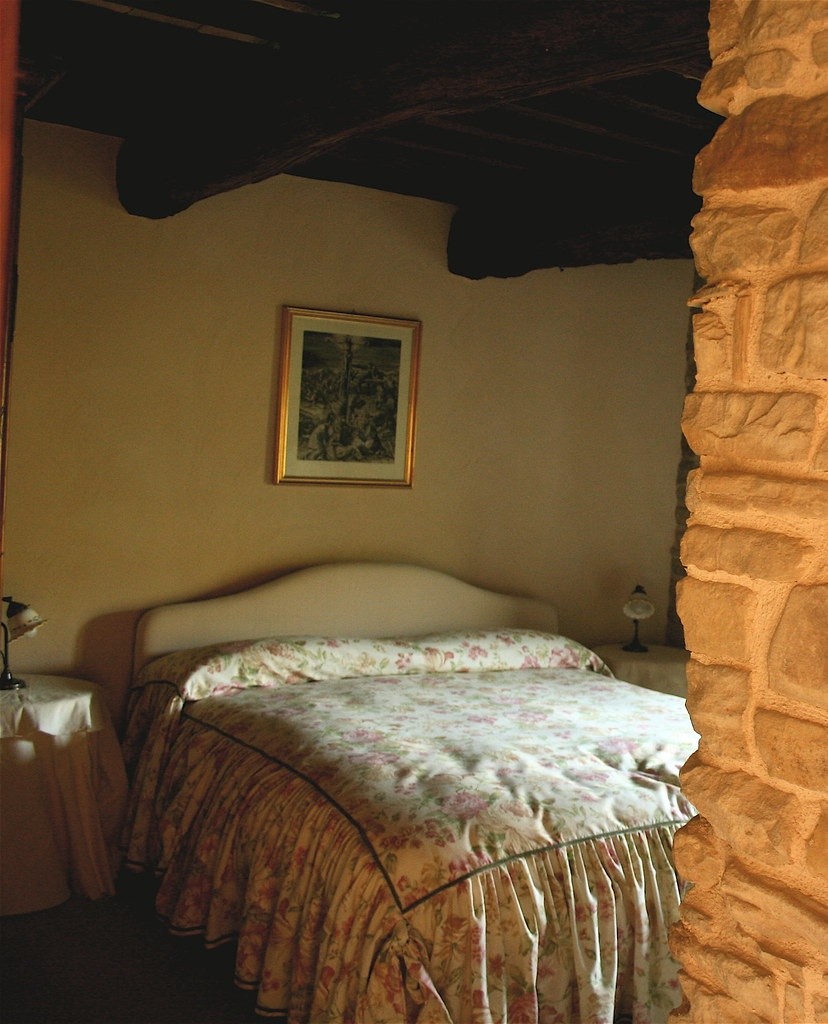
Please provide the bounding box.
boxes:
[273,306,423,489]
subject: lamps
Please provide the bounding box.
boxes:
[0,596,48,690]
[622,583,653,654]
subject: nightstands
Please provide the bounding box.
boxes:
[0,676,129,917]
[594,643,688,695]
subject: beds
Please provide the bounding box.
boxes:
[124,563,702,1024]
